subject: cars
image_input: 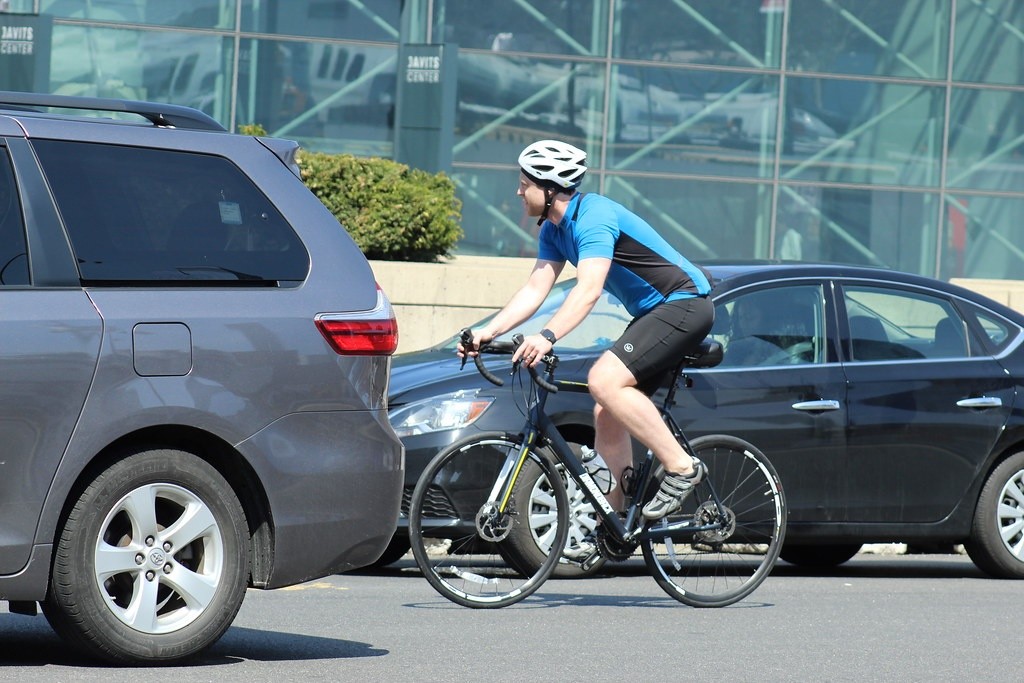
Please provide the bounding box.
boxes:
[366,260,1024,580]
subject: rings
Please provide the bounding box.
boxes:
[529,355,534,360]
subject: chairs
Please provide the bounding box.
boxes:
[847,316,896,361]
[925,320,979,357]
[159,202,224,283]
[773,305,820,370]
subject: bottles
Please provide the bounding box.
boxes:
[581,444,617,494]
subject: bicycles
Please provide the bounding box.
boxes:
[409,328,788,607]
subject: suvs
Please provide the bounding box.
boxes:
[0,93,407,663]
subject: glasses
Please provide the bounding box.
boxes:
[738,311,757,320]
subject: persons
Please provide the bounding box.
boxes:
[722,287,815,366]
[457,139,716,547]
[492,30,516,52]
[776,218,803,261]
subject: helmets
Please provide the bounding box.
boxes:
[518,140,588,192]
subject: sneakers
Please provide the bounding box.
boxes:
[641,456,709,520]
[563,530,597,563]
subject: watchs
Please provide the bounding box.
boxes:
[540,329,557,344]
